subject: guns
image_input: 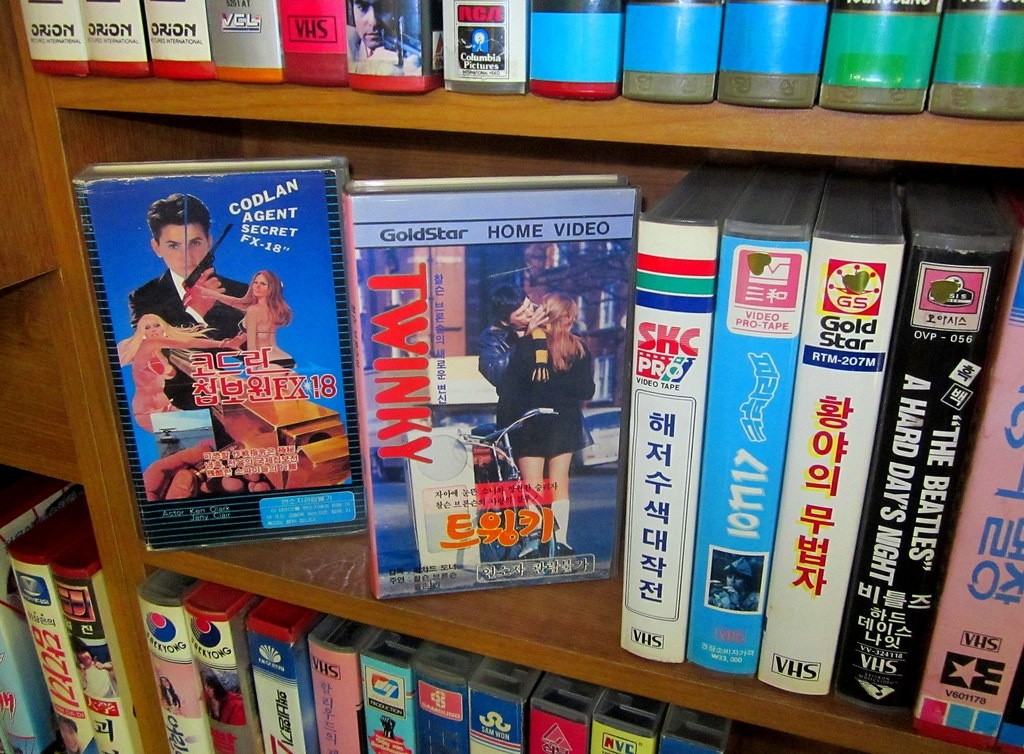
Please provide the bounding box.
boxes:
[380,27,422,59]
[182,223,234,289]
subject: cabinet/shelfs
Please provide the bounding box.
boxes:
[0,0,1024,754]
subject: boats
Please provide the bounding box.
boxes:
[159,430,179,444]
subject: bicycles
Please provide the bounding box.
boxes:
[458,407,558,575]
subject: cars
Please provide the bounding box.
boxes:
[571,407,621,470]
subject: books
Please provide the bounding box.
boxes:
[21,0,1024,119]
[0,481,793,754]
[72,154,1024,754]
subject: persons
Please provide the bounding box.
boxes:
[117,195,290,451]
[477,285,596,453]
[202,669,246,725]
[498,293,596,557]
[70,636,116,701]
[159,674,182,716]
[709,558,760,612]
[348,2,420,77]
[197,270,297,376]
[117,313,247,436]
